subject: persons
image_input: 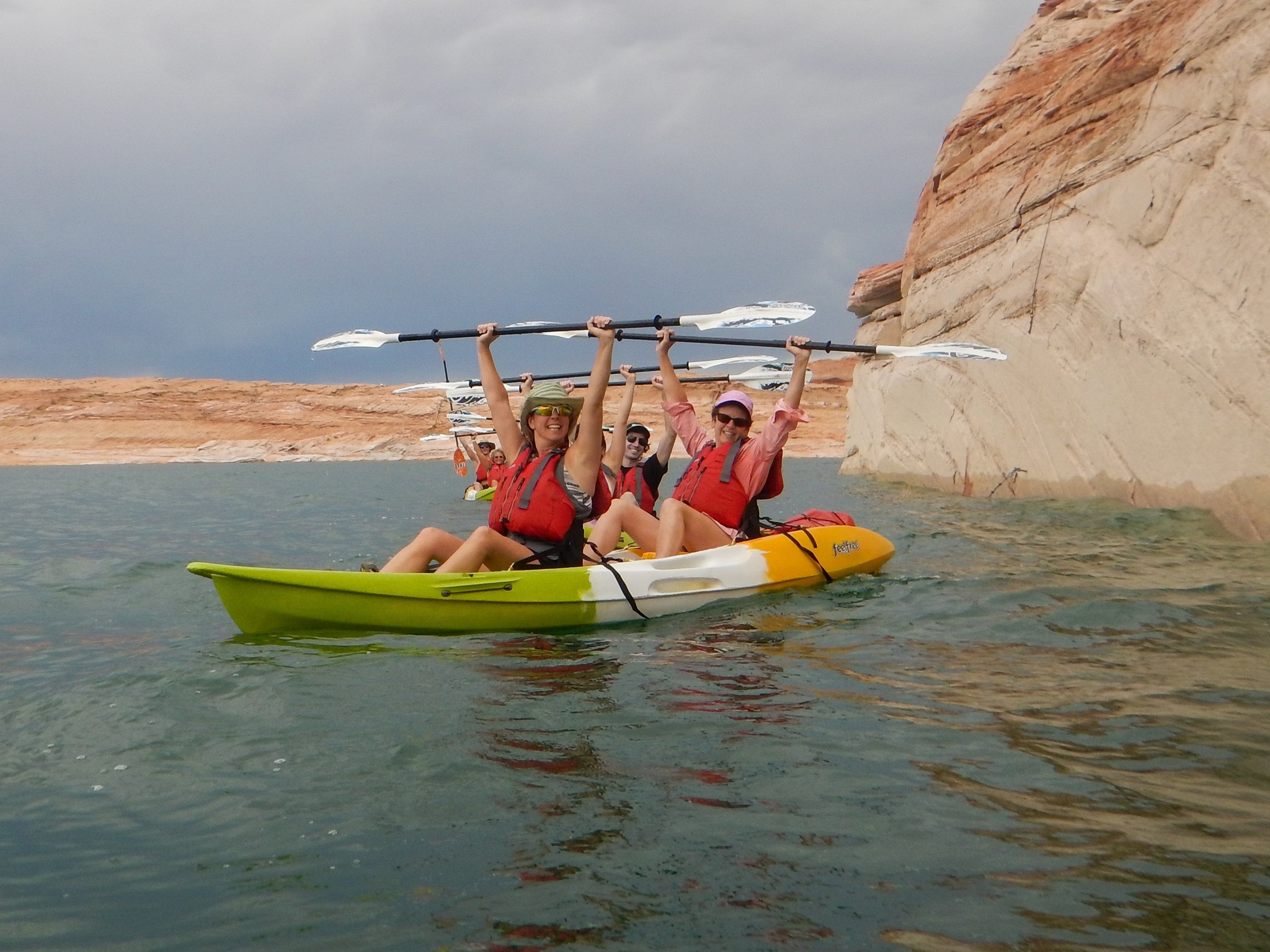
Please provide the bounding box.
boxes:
[583,336,814,566]
[377,315,679,574]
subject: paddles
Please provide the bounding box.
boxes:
[447,410,653,435]
[438,339,467,477]
[449,426,496,433]
[446,362,813,407]
[392,355,778,394]
[310,300,817,352]
[419,432,497,443]
[505,321,1009,362]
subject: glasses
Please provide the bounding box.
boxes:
[495,456,502,458]
[480,445,489,449]
[716,413,750,427]
[626,435,647,446]
[529,405,573,416]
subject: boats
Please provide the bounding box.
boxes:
[463,484,498,501]
[185,525,897,638]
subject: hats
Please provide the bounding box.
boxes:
[715,390,753,417]
[474,439,495,453]
[627,422,650,439]
[519,381,584,442]
